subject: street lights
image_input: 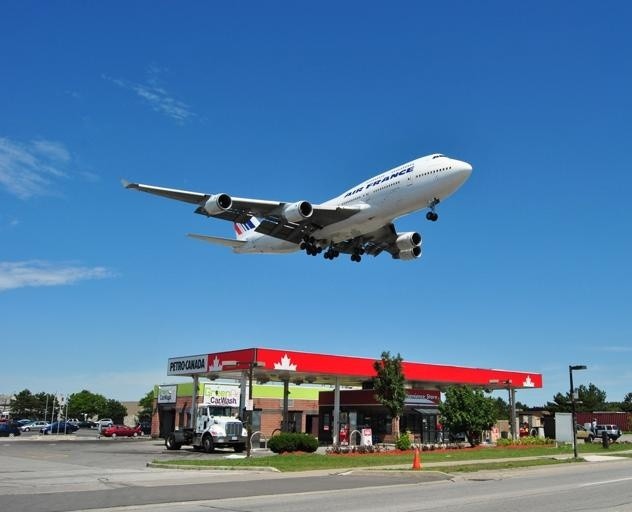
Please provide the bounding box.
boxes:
[569,365,587,457]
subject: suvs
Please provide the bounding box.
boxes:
[165,403,248,452]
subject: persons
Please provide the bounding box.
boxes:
[591,418,598,437]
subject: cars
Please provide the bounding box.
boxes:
[577,424,622,442]
[103,422,151,437]
[0,418,113,437]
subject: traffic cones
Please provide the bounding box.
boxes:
[412,448,422,471]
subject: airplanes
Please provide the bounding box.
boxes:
[124,153,473,262]
